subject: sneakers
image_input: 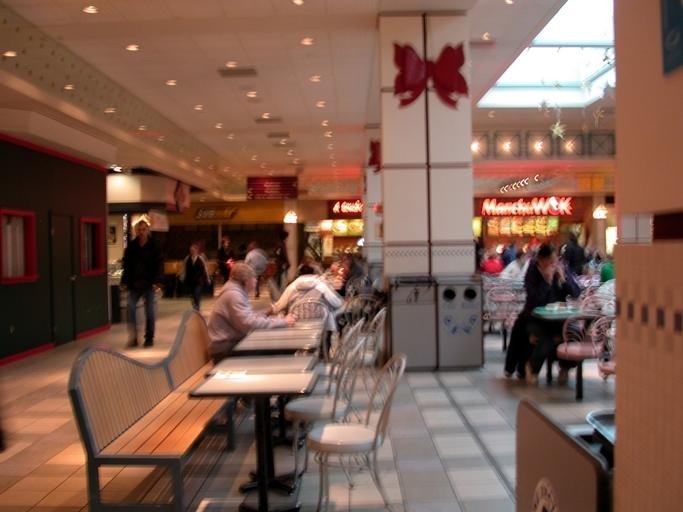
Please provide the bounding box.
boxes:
[526,361,539,385]
[557,367,568,385]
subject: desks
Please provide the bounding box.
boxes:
[534,302,604,318]
[204,354,319,494]
[231,339,317,356]
[187,372,319,510]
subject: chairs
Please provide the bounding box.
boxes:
[303,353,406,511]
[286,337,368,487]
[289,298,330,363]
[247,328,323,339]
[555,313,608,400]
[353,307,387,408]
[591,316,615,375]
[293,317,365,454]
[484,287,519,350]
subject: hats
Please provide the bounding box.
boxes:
[230,263,256,283]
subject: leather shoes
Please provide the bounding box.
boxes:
[143,339,153,346]
[123,341,139,349]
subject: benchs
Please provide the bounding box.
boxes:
[66,309,239,511]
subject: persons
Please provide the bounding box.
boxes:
[560,231,600,274]
[503,242,584,386]
[272,245,290,293]
[177,243,212,313]
[216,232,239,285]
[244,241,269,299]
[206,263,297,412]
[119,220,164,351]
[473,234,545,274]
